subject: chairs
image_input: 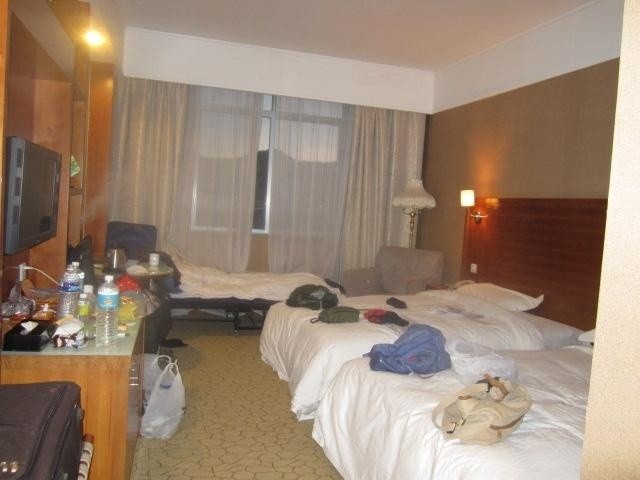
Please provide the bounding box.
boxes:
[107,221,345,331]
[340,246,444,295]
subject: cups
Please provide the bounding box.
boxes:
[149,253,160,265]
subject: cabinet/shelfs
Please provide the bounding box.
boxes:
[1,318,146,476]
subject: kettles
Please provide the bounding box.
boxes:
[102,242,129,275]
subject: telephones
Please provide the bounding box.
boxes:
[453,279,476,289]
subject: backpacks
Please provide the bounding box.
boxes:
[286,284,338,309]
[363,323,451,374]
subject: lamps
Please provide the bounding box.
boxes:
[460,190,489,224]
[394,180,436,247]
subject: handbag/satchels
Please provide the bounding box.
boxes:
[311,307,359,322]
[432,374,532,444]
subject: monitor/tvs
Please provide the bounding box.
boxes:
[3,136,63,255]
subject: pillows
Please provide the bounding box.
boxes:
[577,328,594,347]
[458,283,543,313]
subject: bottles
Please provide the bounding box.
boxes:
[59,265,79,316]
[71,262,84,292]
[97,275,119,349]
[84,285,96,308]
[76,293,90,323]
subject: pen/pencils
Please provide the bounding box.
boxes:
[117,333,131,337]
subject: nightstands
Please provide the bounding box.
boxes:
[428,283,450,291]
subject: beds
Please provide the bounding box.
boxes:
[311,342,591,476]
[259,291,592,421]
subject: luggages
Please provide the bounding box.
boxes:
[0,382,84,480]
